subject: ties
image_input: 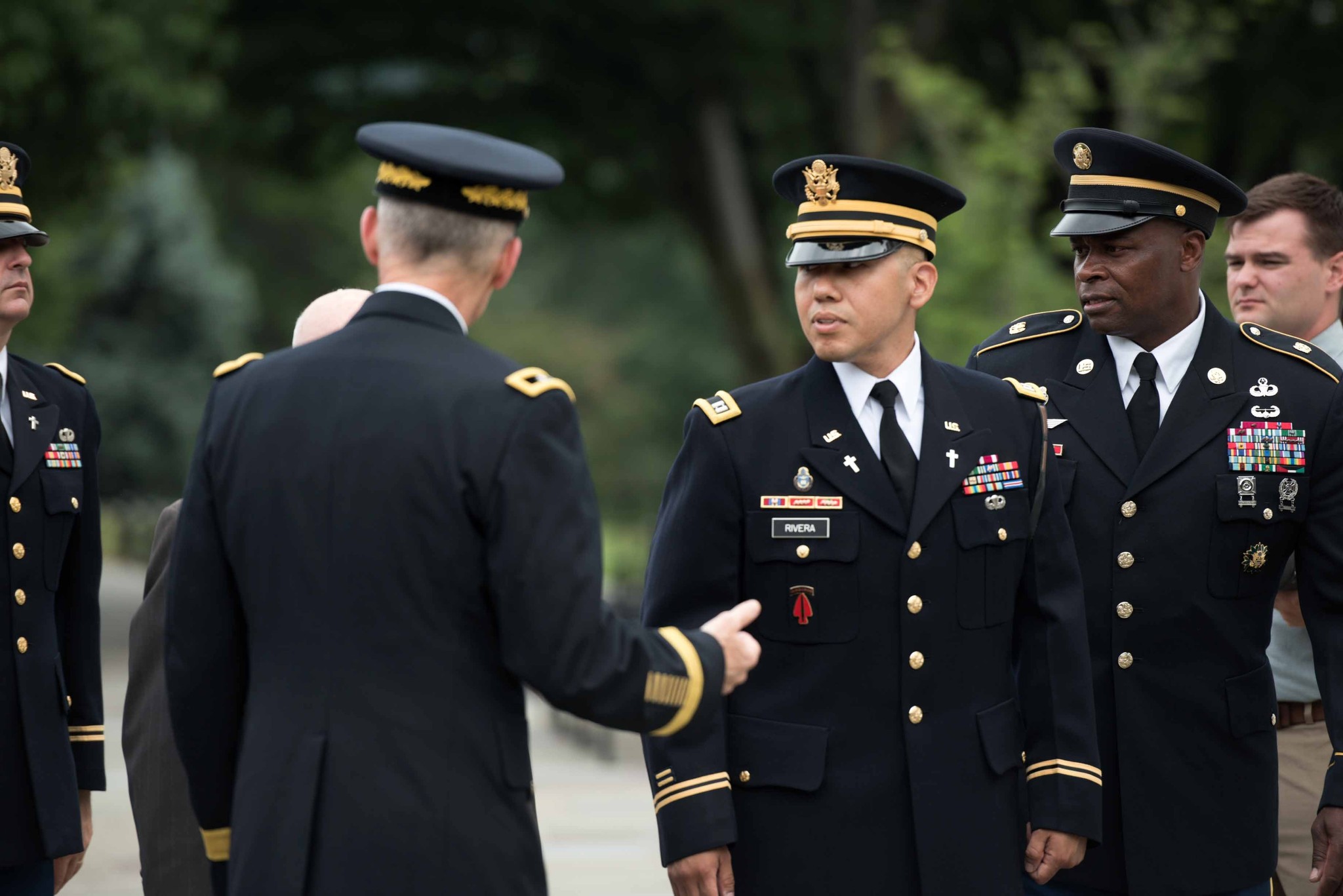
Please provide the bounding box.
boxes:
[1124,353,1160,463]
[869,381,918,525]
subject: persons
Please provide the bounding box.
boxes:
[0,144,106,896]
[1224,173,1343,896]
[120,288,374,896]
[965,127,1343,896]
[639,153,1104,896]
[166,122,761,896]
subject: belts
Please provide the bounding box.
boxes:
[1275,701,1326,729]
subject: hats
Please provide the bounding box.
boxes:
[355,120,564,223]
[772,153,966,268]
[0,141,49,248]
[1050,127,1249,240]
